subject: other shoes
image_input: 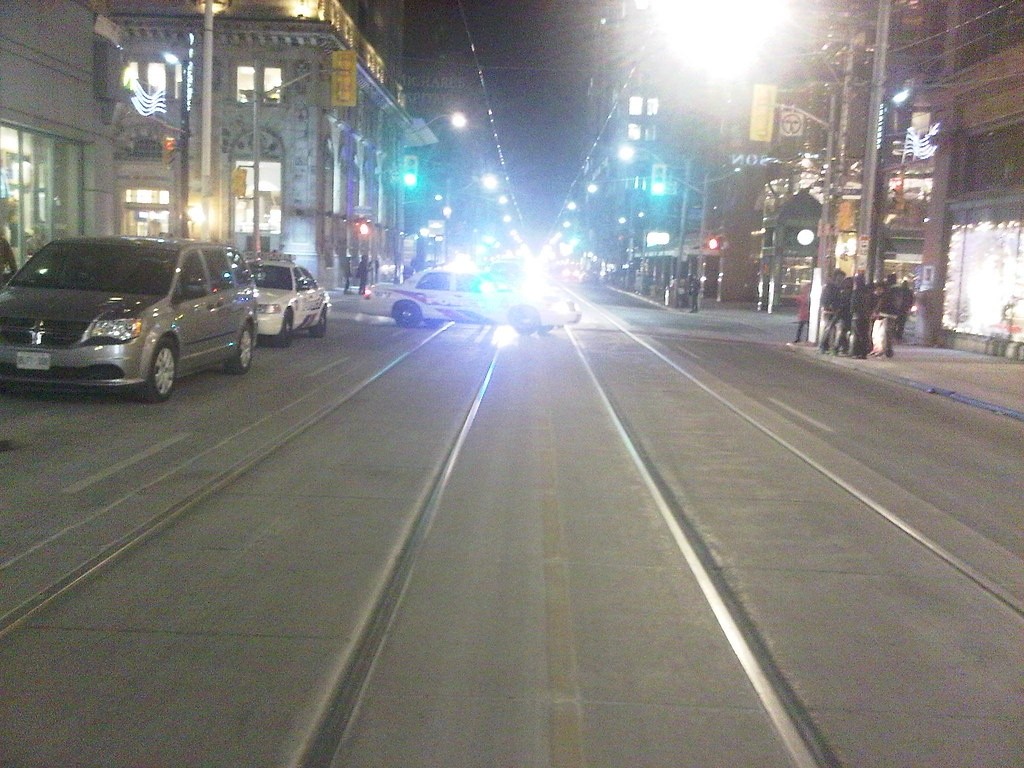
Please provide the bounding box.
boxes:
[852,356,867,362]
[837,351,849,357]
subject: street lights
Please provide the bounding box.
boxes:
[163,52,189,239]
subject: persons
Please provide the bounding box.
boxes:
[355,255,379,295]
[343,254,353,294]
[689,274,701,314]
[794,270,914,359]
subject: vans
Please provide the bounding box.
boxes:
[0,235,259,404]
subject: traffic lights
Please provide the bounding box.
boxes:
[709,239,718,250]
[651,163,666,196]
[360,225,369,235]
[405,155,417,189]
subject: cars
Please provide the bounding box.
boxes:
[356,267,583,335]
[244,261,334,349]
